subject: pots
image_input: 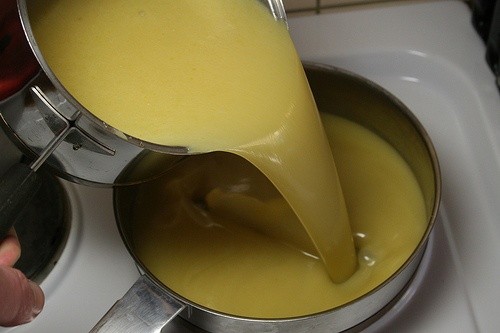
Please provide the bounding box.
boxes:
[0,0,290,247]
[83,60,443,333]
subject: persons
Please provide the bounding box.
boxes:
[0,227,45,327]
